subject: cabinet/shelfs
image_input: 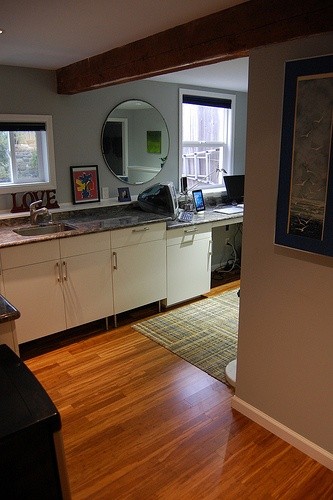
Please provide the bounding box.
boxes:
[0,220,214,344]
[0,344,73,500]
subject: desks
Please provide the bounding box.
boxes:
[161,197,245,308]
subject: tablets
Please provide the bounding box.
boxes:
[192,189,205,211]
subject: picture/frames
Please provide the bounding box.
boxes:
[71,165,100,204]
[271,54,333,257]
[118,188,131,202]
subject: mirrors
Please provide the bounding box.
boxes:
[101,98,170,185]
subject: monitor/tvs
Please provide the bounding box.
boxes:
[223,174,244,205]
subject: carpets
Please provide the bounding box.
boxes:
[132,287,240,387]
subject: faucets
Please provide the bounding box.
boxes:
[29,200,47,225]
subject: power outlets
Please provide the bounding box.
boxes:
[225,238,231,247]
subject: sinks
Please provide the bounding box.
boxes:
[12,223,79,236]
